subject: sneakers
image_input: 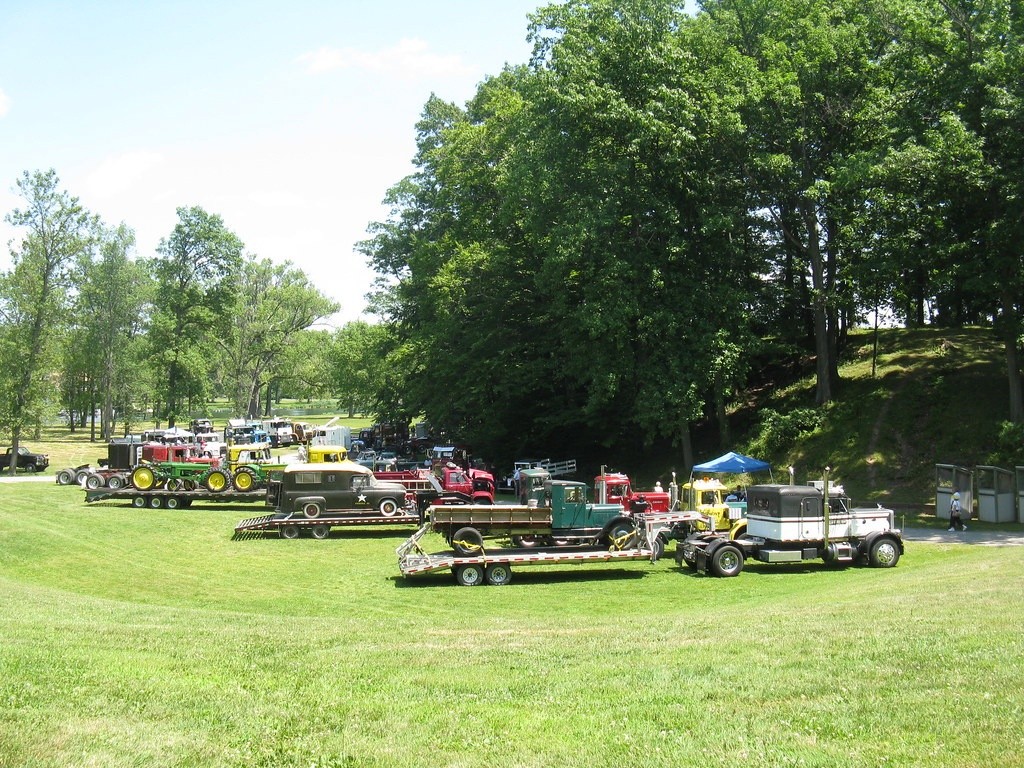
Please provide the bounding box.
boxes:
[962,525,967,531]
[948,528,955,532]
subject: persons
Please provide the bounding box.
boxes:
[725,490,738,502]
[734,485,744,501]
[949,486,960,531]
[487,463,498,492]
[947,492,967,532]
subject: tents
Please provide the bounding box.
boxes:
[689,451,777,485]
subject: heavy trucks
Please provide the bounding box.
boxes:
[55,412,907,589]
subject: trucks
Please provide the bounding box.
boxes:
[0,447,50,474]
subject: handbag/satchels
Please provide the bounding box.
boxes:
[952,510,962,517]
